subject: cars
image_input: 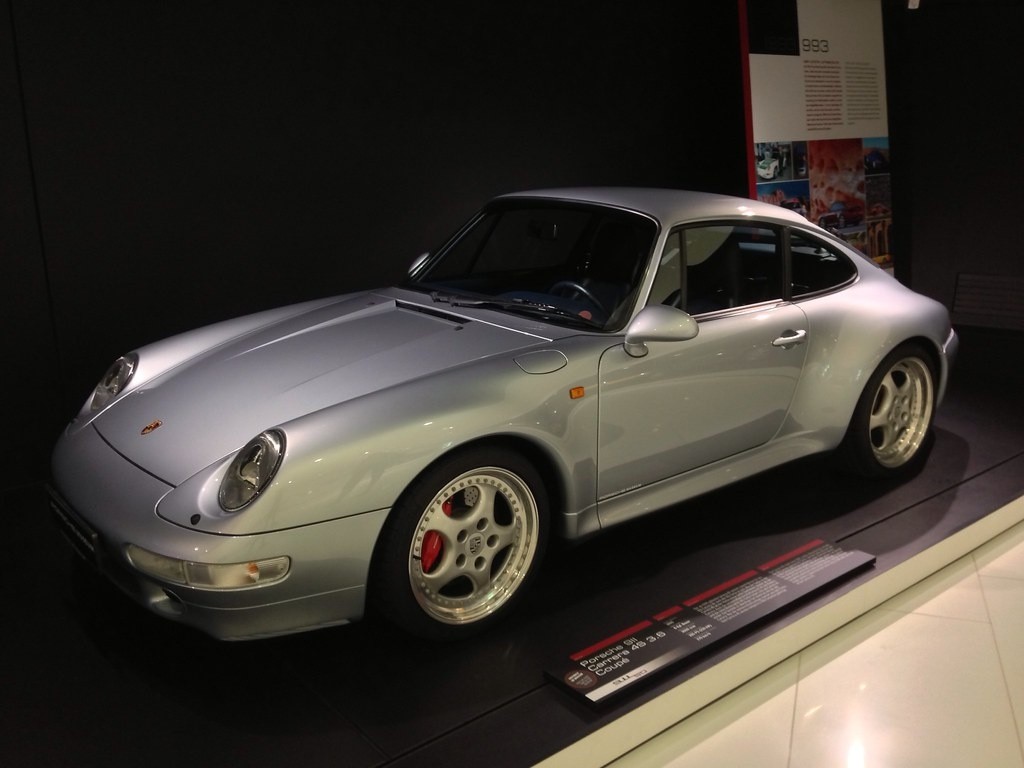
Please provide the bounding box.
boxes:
[43,186,958,642]
[817,200,864,229]
[757,151,786,180]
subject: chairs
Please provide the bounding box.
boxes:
[578,218,636,317]
[680,244,730,315]
